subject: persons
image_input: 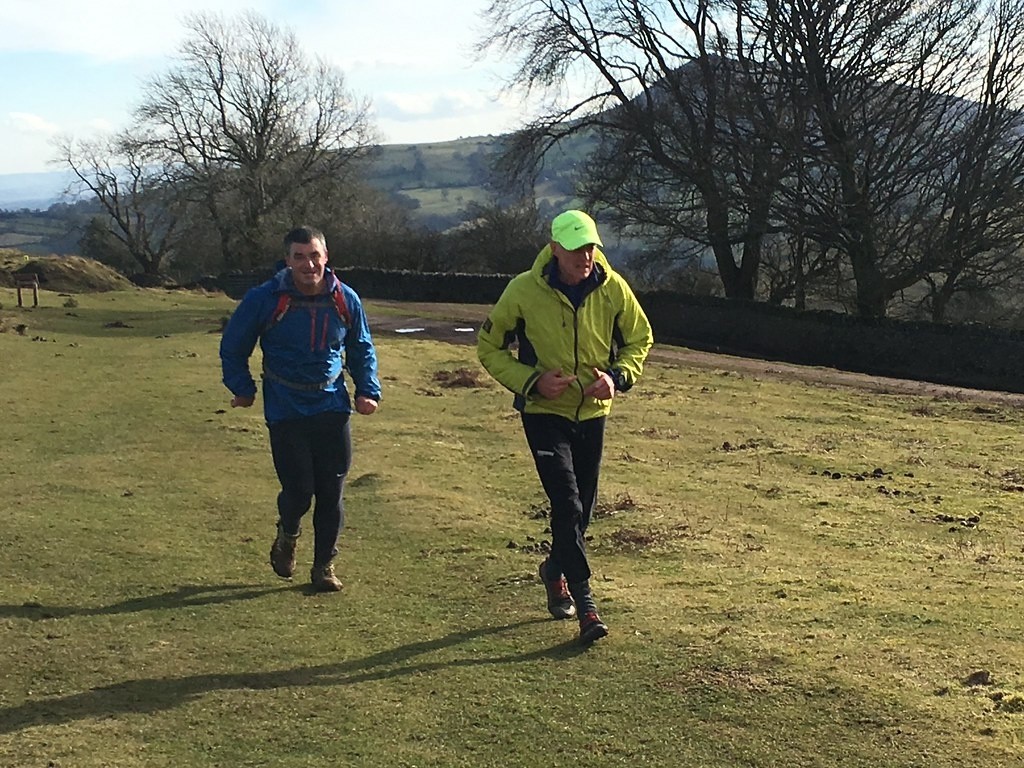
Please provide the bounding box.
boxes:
[477,210,654,640]
[220,224,381,589]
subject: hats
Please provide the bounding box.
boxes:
[551,210,603,251]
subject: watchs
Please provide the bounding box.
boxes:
[605,368,625,391]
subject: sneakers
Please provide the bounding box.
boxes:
[270,520,302,578]
[311,561,343,591]
[539,560,576,618]
[580,611,609,645]
